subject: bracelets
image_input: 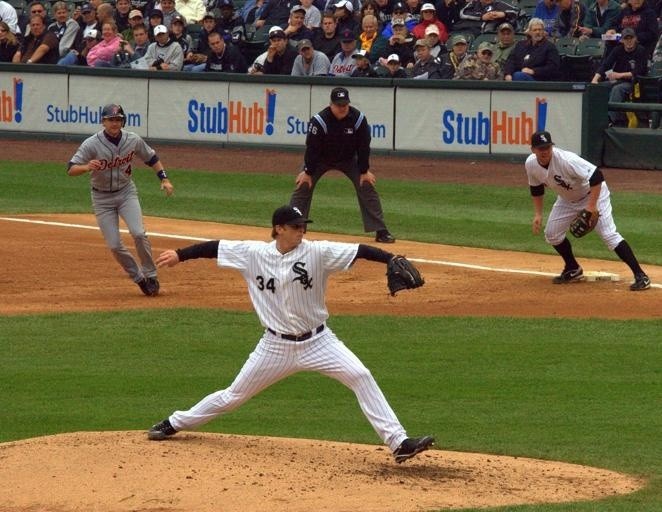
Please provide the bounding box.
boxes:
[156,169,168,181]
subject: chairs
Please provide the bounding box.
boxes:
[186,22,203,52]
[450,0,607,63]
[636,61,662,103]
[5,0,87,19]
[243,24,274,52]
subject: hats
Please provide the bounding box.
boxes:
[204,13,215,21]
[330,87,350,105]
[149,10,162,18]
[273,205,314,225]
[128,10,144,19]
[219,1,233,9]
[532,132,556,148]
[269,1,515,63]
[172,15,185,25]
[80,4,94,14]
[154,26,168,37]
[622,28,635,39]
[85,29,102,40]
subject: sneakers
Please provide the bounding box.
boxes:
[376,229,395,242]
[138,277,159,296]
[552,263,584,284]
[148,418,178,441]
[393,435,435,462]
[630,273,651,291]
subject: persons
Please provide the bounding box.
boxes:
[147,206,436,465]
[593,28,651,127]
[523,130,652,292]
[1,0,661,84]
[65,103,175,295]
[287,85,397,245]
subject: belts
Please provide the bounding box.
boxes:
[267,325,325,341]
[91,186,122,195]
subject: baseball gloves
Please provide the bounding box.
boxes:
[569,209,599,238]
[386,255,424,296]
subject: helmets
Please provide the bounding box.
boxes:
[101,103,126,119]
